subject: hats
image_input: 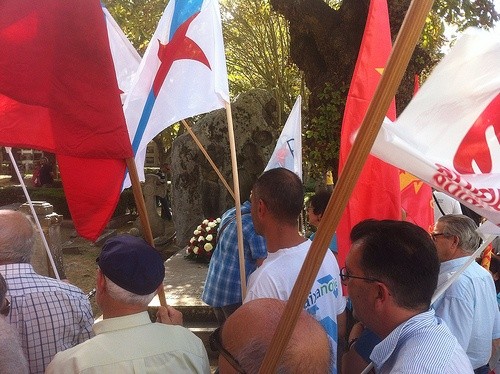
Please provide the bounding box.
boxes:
[96,234,165,294]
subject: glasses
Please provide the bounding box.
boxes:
[209,328,247,374]
[0,299,11,316]
[429,232,450,241]
[339,266,392,297]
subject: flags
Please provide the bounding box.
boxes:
[122,0,229,158]
[103,8,147,193]
[0,0,132,242]
[265,94,302,180]
[337,0,500,296]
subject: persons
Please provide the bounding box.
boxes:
[249,167,347,374]
[201,198,268,321]
[306,189,338,252]
[0,210,96,374]
[32,157,54,188]
[434,215,500,374]
[0,315,29,374]
[209,298,334,374]
[45,238,211,374]
[339,217,474,374]
